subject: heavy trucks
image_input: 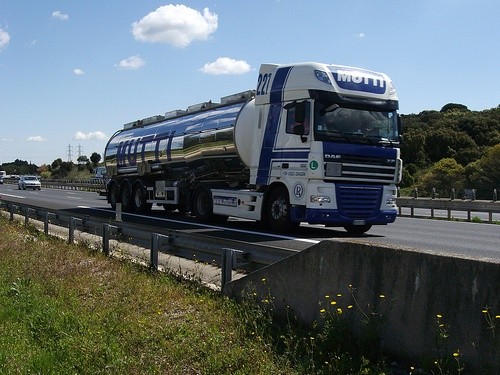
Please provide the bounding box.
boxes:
[102,61,404,236]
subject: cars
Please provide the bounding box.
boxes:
[18,175,41,191]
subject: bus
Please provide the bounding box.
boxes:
[93,167,106,178]
